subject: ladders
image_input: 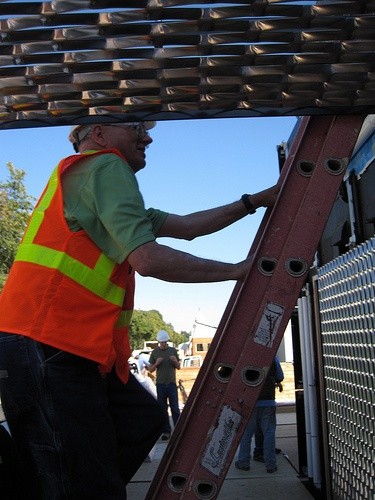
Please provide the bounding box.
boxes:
[146,112,367,500]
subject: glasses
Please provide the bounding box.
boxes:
[104,121,149,137]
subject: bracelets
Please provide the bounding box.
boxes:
[240,193,257,215]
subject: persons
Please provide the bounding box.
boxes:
[132,330,181,440]
[235,353,284,472]
[0,121,280,500]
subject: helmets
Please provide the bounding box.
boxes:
[158,330,170,342]
[68,120,157,143]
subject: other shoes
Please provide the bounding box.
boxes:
[236,460,250,470]
[268,466,277,473]
[252,454,264,462]
[274,448,281,454]
